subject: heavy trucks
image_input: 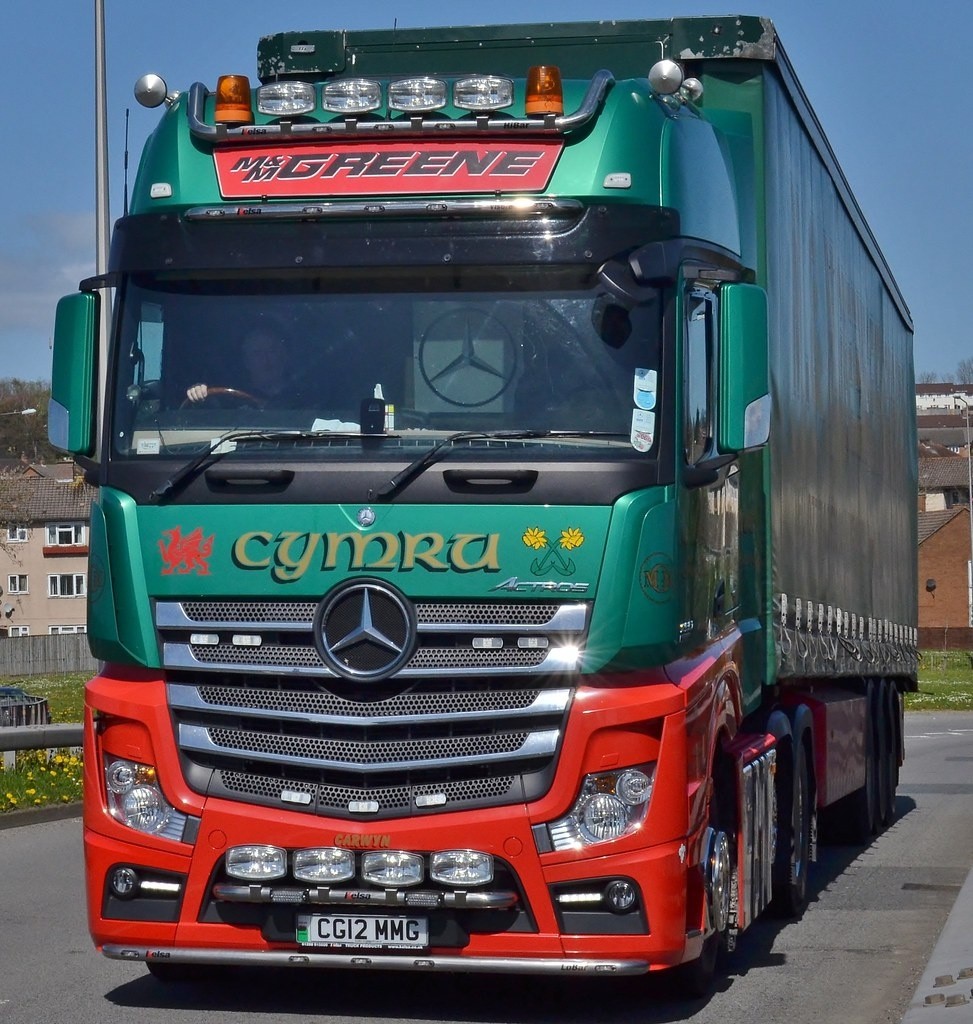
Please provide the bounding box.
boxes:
[48,13,918,1002]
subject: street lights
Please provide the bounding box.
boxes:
[952,395,973,604]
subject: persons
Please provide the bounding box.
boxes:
[186,321,327,412]
